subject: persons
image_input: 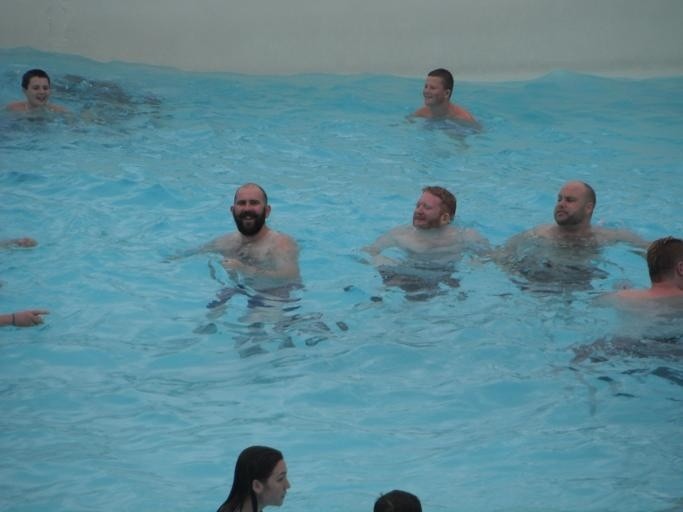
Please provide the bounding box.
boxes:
[604,238,683,306]
[374,490,421,511]
[8,70,77,118]
[0,308,48,328]
[365,185,494,283]
[216,445,290,511]
[496,181,654,288]
[409,68,479,133]
[157,183,300,294]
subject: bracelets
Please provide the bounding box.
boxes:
[8,312,14,323]
